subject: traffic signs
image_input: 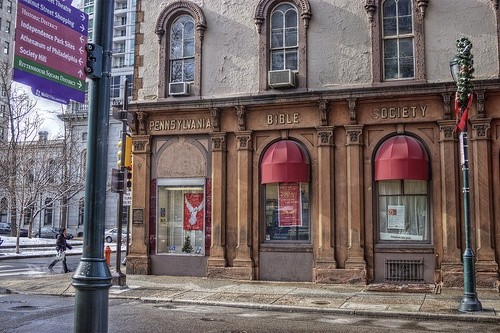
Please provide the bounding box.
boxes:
[10,1,89,105]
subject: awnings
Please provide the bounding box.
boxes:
[375,135,429,181]
[261,140,310,184]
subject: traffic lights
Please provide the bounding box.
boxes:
[117,137,132,167]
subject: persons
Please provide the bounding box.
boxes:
[48,227,72,273]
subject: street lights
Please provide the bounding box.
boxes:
[447,54,482,313]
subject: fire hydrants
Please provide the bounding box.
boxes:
[104,245,112,267]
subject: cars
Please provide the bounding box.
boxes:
[104,228,131,243]
[0,222,29,236]
[32,227,73,240]
